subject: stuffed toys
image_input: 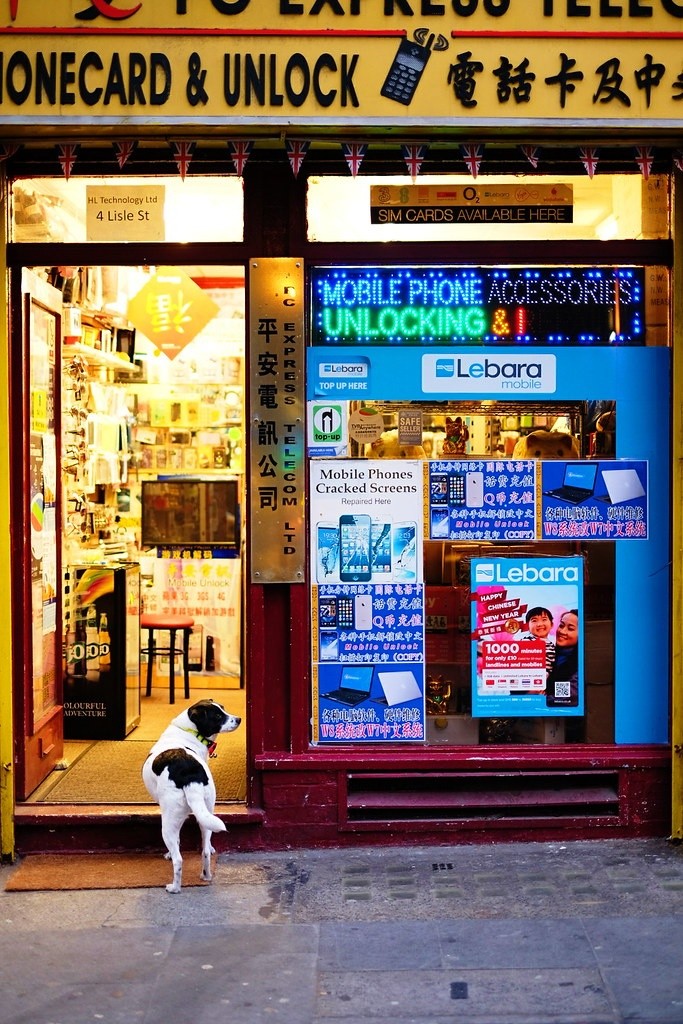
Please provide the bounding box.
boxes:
[513,431,580,459]
[364,427,428,460]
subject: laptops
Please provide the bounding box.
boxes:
[320,664,376,707]
[542,462,599,505]
[369,671,423,706]
[594,470,645,505]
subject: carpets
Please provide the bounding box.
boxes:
[35,740,246,802]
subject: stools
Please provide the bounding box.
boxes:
[140,612,195,705]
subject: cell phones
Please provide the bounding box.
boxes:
[157,624,222,677]
[319,594,373,662]
[317,514,418,585]
[431,471,484,539]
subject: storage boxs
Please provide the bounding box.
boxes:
[63,308,81,344]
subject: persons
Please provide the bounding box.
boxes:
[519,607,556,680]
[547,609,579,707]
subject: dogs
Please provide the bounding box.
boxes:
[141,698,242,896]
